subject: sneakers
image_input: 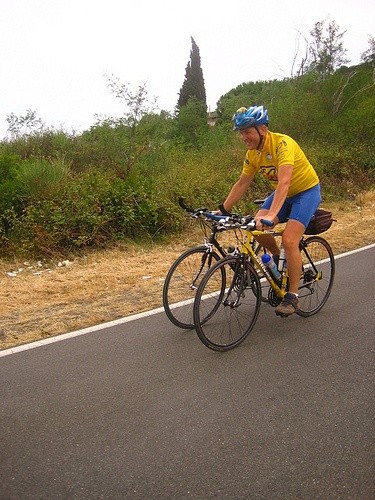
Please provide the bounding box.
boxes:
[275,293,299,316]
[272,255,279,265]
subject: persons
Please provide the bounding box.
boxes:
[212,105,321,317]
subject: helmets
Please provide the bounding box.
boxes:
[231,104,269,131]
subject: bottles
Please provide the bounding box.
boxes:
[227,247,237,256]
[278,245,286,271]
[261,254,281,280]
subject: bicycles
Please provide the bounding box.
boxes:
[163,196,337,352]
